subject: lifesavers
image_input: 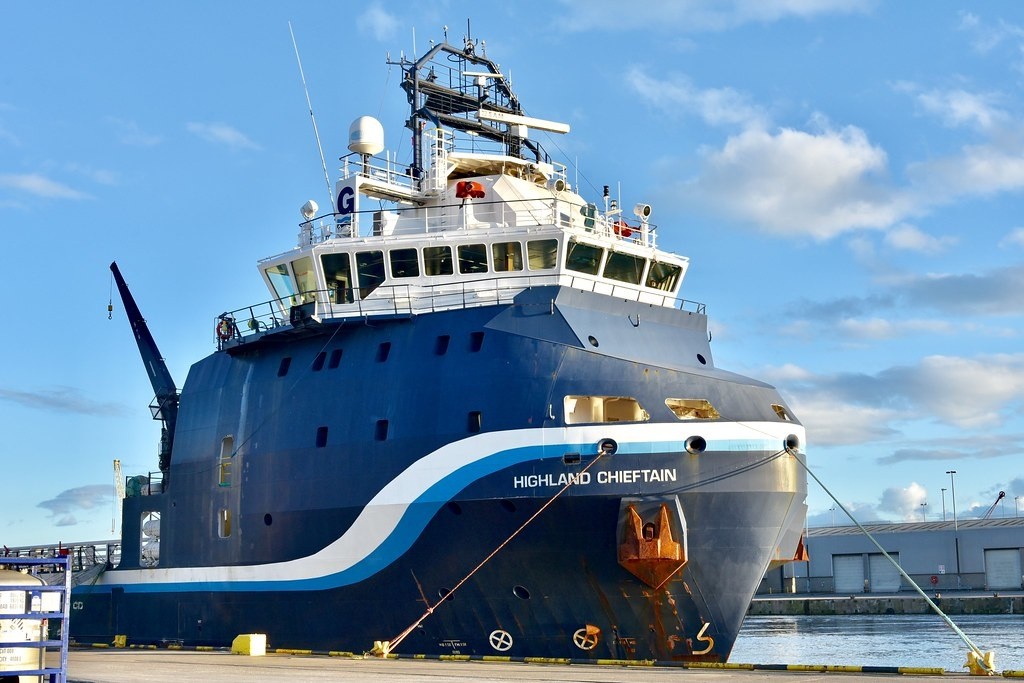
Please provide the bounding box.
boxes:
[217,321,232,339]
[931,576,937,583]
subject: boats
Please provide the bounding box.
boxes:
[46,6,813,670]
